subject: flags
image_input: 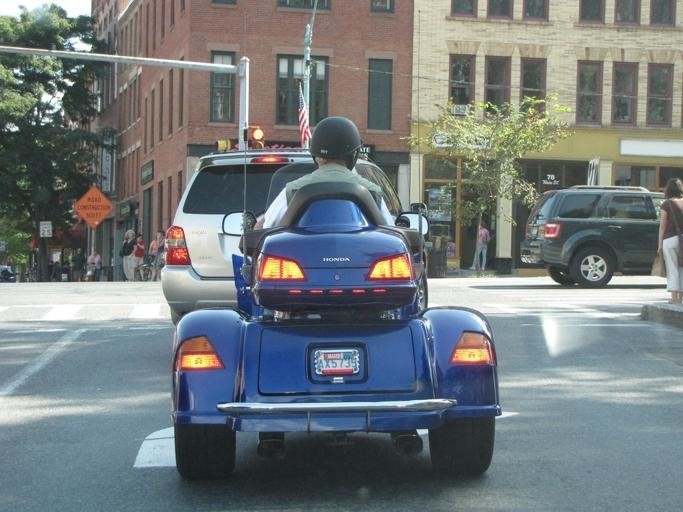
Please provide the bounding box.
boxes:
[297,86,311,147]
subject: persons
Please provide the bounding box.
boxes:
[469,222,490,270]
[48,229,165,282]
[396,216,410,228]
[253,116,396,228]
[658,178,683,304]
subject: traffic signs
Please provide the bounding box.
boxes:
[39,221,52,238]
[74,188,111,228]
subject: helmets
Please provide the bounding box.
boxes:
[307,116,362,169]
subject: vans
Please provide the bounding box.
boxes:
[520,184,667,287]
[161,148,428,324]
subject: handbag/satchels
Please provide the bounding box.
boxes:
[652,255,666,277]
[677,236,683,267]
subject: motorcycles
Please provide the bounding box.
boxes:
[171,162,499,480]
